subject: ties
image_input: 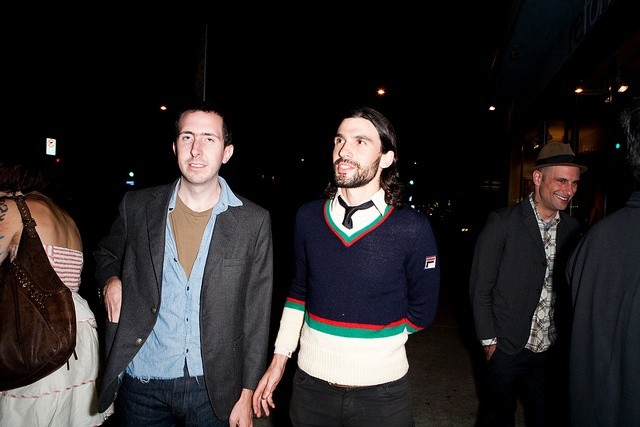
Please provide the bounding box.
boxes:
[337,195,374,229]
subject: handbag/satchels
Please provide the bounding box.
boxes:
[1,196,78,391]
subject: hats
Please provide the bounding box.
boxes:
[529,142,588,173]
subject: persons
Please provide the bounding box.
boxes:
[565,106,638,425]
[253,107,439,427]
[469,139,591,425]
[1,159,115,427]
[84,107,275,427]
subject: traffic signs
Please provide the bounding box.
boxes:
[46,138,56,155]
